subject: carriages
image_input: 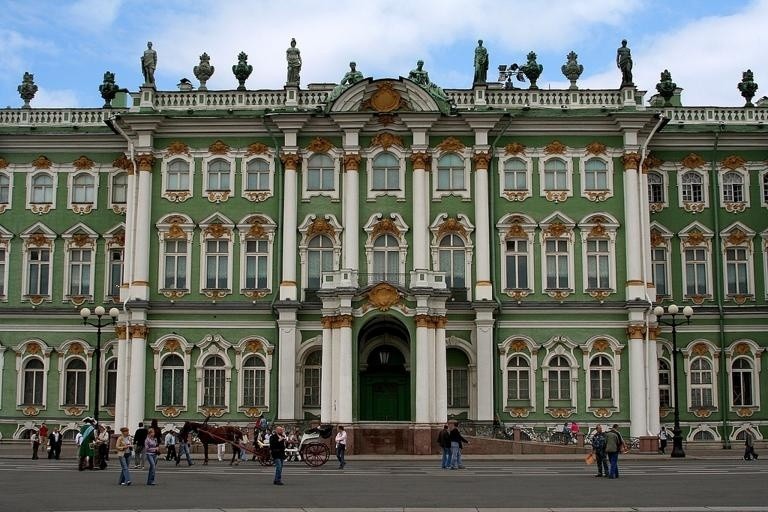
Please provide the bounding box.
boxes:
[177,420,333,467]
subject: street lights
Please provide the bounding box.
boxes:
[652,304,694,458]
[80,306,120,423]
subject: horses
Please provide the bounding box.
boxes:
[180,420,243,466]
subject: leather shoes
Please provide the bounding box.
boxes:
[595,473,619,479]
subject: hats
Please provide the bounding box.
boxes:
[82,417,97,425]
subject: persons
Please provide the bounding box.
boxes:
[269,425,286,485]
[217,443,225,462]
[474,40,490,85]
[449,420,469,471]
[561,417,759,479]
[237,415,302,462]
[135,422,147,468]
[436,424,452,471]
[115,426,135,486]
[144,427,163,486]
[165,430,178,461]
[139,40,158,85]
[616,38,634,87]
[151,419,161,449]
[176,430,194,466]
[78,417,110,470]
[406,59,454,104]
[335,426,347,469]
[31,423,62,459]
[320,62,363,104]
[286,36,303,83]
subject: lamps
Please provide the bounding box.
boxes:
[495,62,527,90]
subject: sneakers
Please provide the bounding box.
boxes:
[120,480,131,486]
